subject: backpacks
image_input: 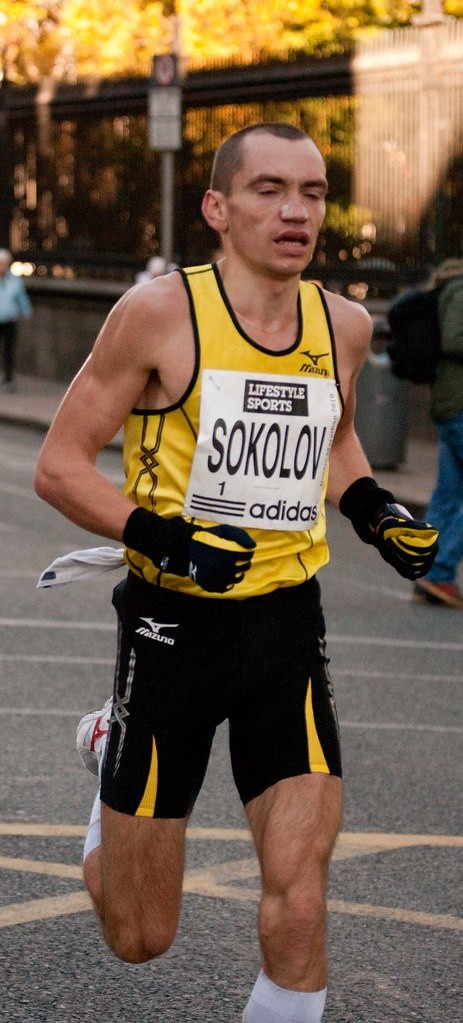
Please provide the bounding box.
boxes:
[386,281,460,386]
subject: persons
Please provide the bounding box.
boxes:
[0,248,33,393]
[32,122,441,1023]
[408,150,463,609]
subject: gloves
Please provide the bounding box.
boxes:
[339,477,439,581]
[122,508,257,595]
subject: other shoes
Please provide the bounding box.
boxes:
[413,578,462,608]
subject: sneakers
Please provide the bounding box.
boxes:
[76,694,114,777]
